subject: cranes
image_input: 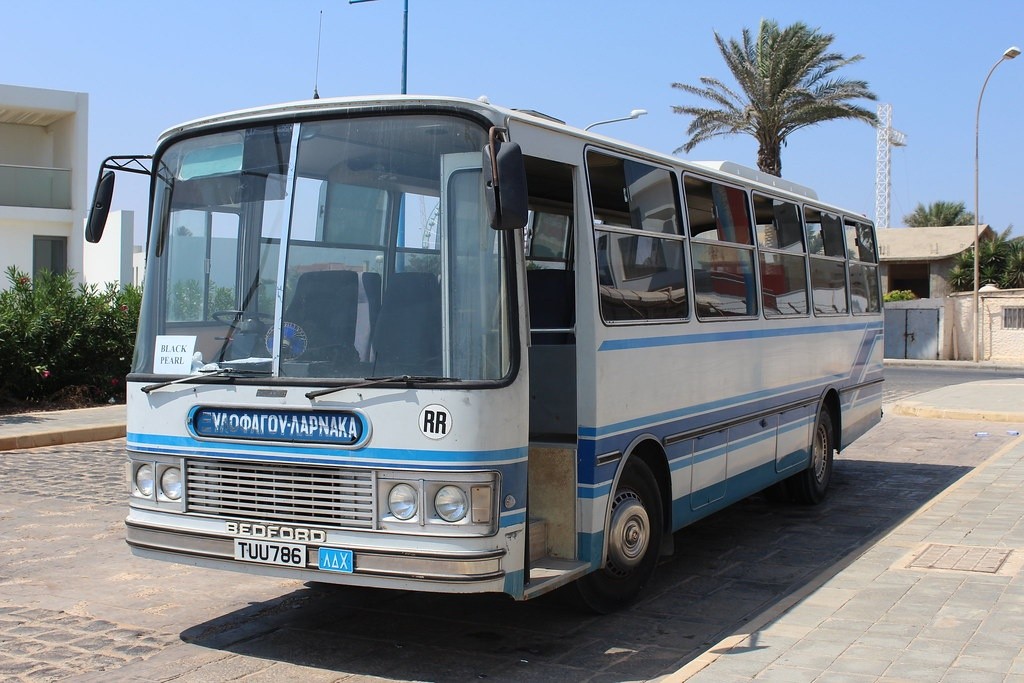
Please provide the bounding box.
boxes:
[875,103,907,229]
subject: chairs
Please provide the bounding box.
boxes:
[258,270,442,377]
[527,267,846,345]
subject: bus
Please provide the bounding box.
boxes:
[84,92,886,616]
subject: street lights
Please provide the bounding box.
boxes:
[582,109,650,132]
[972,45,1021,367]
